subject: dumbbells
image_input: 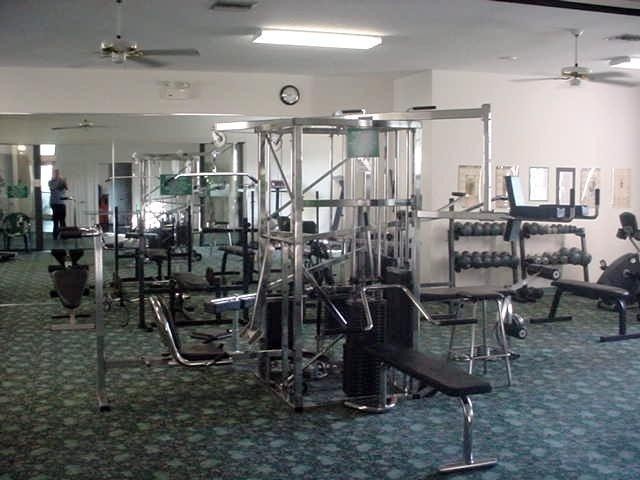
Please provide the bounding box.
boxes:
[452,220,592,270]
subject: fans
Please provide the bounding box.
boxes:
[63,0,200,70]
[49,117,123,131]
[508,25,639,88]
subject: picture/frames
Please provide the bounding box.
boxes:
[556,166,576,209]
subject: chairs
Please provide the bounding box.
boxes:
[0,212,32,251]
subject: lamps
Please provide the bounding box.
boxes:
[253,29,384,51]
[610,56,640,71]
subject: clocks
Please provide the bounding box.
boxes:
[280,85,301,105]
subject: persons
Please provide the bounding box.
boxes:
[48,168,68,241]
[258,216,310,297]
